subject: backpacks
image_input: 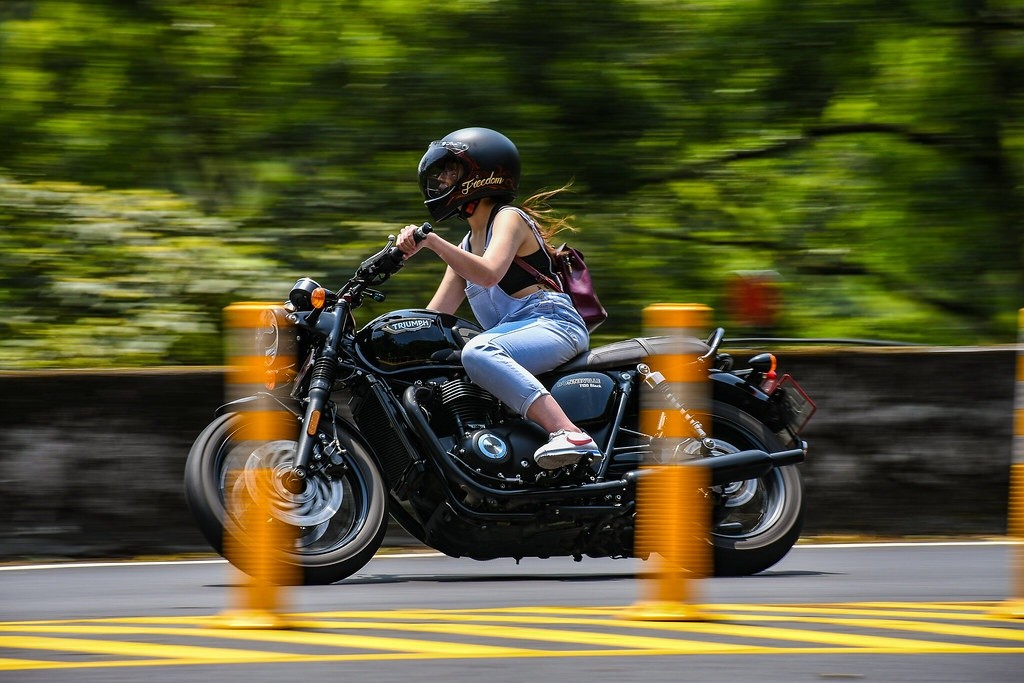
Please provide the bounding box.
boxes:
[490,205,609,323]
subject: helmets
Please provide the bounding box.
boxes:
[417,126,521,223]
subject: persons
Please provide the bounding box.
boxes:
[396,127,601,468]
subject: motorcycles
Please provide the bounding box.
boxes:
[183,221,807,588]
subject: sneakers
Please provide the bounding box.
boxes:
[533,428,603,468]
